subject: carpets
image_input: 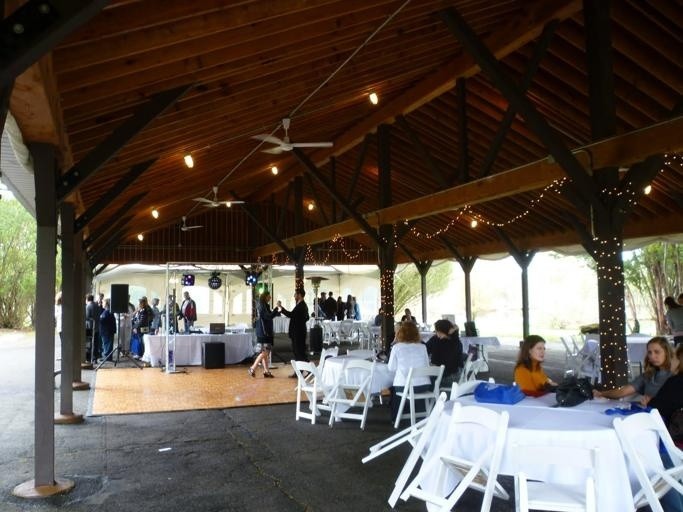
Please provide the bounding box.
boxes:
[88,361,393,417]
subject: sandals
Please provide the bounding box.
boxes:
[248,367,274,378]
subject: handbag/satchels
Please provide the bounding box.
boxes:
[140,326,149,333]
[475,383,526,404]
[556,376,593,406]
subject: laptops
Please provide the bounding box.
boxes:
[208,323,225,334]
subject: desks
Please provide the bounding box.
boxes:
[139,333,272,367]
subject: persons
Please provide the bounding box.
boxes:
[678,293,683,306]
[663,296,682,357]
[513,334,557,398]
[247,287,363,379]
[374,307,464,425]
[591,335,672,398]
[55,288,198,363]
[638,343,683,512]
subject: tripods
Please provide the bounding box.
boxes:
[95,316,143,369]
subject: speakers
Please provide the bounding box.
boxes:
[201,341,225,368]
[111,284,128,313]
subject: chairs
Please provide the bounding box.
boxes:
[362,373,683,512]
[560,328,681,385]
[290,316,501,432]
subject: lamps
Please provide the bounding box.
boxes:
[152,208,159,218]
[183,152,194,168]
[369,91,379,105]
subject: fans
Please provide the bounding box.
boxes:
[180,216,204,232]
[192,186,246,208]
[250,118,334,154]
